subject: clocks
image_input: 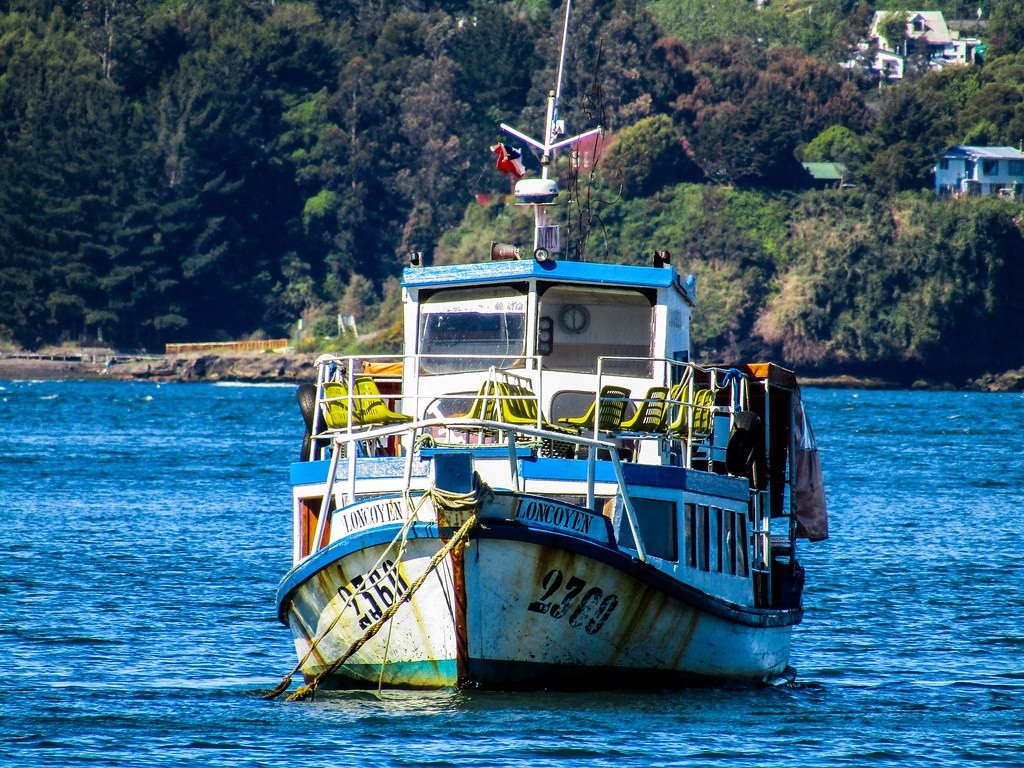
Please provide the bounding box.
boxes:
[559,305,590,334]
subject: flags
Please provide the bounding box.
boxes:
[572,128,619,174]
[489,126,541,180]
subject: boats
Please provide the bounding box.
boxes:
[275,1,809,696]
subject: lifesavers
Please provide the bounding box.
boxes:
[300,427,321,461]
[297,384,331,448]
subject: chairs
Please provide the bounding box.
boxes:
[319,378,414,459]
[436,380,716,472]
